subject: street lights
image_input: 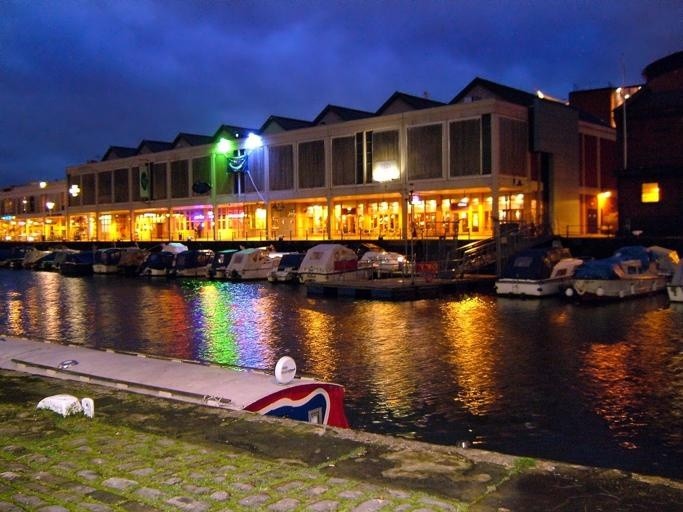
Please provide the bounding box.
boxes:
[46,198,55,239]
[215,132,263,201]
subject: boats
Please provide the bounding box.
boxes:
[0,329,350,431]
[491,225,683,308]
